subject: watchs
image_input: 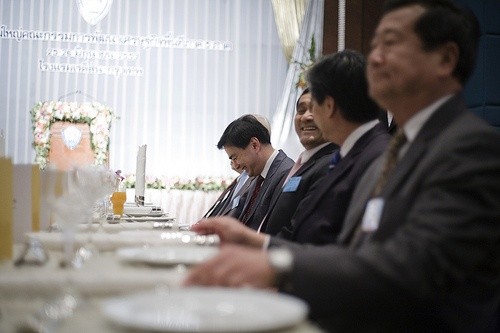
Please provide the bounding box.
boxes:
[269,246,294,294]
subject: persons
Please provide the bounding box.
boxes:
[181,0,500,332]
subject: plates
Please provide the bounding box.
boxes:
[117,245,222,264]
[120,217,173,221]
[101,287,307,333]
[126,212,163,217]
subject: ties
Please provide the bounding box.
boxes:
[241,175,265,223]
[352,130,408,235]
[215,175,240,203]
[329,148,342,173]
[281,152,304,189]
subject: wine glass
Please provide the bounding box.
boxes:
[38,167,117,278]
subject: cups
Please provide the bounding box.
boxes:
[112,184,127,217]
